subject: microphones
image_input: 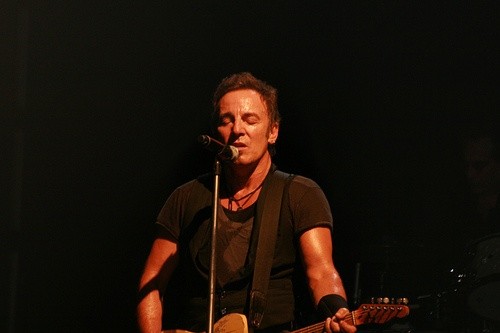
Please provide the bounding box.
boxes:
[199,134,239,160]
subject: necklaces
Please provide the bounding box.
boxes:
[228,179,265,211]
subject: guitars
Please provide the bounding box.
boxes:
[197,297,410,333]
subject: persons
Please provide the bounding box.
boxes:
[137,71,357,333]
